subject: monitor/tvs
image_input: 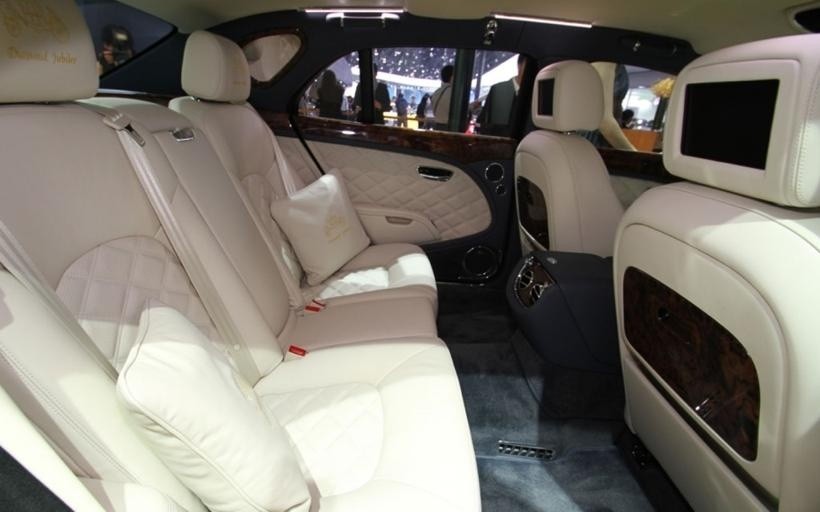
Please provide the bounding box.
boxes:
[680,79,780,171]
[537,78,554,117]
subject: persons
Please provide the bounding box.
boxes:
[315,54,528,137]
[96,24,135,77]
[621,110,633,128]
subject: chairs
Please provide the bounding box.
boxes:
[506,57,627,269]
[98,85,443,357]
[1,0,490,512]
[165,23,444,310]
[612,29,820,512]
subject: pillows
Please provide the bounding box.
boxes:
[270,163,371,287]
[113,292,315,512]
